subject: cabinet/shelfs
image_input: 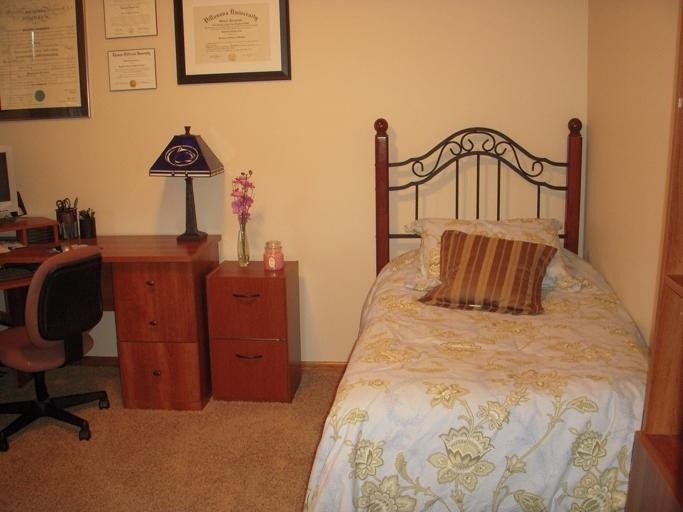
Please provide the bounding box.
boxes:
[620,1,682,511]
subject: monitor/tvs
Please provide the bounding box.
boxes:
[0,146,19,219]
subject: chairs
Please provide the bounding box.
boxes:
[1,246,112,453]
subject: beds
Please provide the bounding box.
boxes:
[298,114,655,512]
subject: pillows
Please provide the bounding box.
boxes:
[415,228,560,316]
[401,215,585,293]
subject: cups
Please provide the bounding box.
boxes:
[79,218,97,239]
[54,208,78,240]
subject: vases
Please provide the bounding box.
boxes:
[235,228,251,268]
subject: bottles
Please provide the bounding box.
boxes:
[263,240,284,272]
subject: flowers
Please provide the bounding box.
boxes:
[229,169,256,226]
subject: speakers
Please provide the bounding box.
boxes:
[16,191,29,215]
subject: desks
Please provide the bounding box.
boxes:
[0,213,226,412]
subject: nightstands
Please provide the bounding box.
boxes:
[203,260,303,405]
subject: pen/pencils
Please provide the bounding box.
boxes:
[61,201,64,211]
[74,197,78,211]
[77,207,95,221]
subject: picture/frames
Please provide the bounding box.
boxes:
[0,2,94,124]
[170,0,293,87]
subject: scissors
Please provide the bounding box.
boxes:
[56,198,71,209]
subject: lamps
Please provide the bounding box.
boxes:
[146,124,226,242]
